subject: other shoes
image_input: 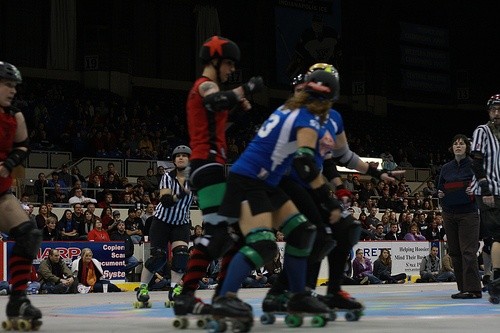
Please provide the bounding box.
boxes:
[360,276,368,285]
[452,292,482,299]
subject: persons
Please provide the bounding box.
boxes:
[0,61,500,333]
[168,36,263,329]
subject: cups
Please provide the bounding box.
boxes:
[144,236,148,242]
[102,284,108,293]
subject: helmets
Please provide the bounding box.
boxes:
[305,69,341,97]
[306,64,338,79]
[199,35,240,62]
[487,94,500,106]
[289,74,306,93]
[172,146,191,158]
[0,61,23,84]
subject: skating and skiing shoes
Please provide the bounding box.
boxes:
[207,294,253,333]
[488,277,500,304]
[164,285,184,308]
[481,274,492,292]
[2,290,42,332]
[259,292,288,325]
[134,283,152,308]
[284,294,332,327]
[326,288,363,322]
[172,292,212,328]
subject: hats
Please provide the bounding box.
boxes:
[113,210,120,216]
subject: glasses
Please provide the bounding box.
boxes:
[489,107,500,113]
